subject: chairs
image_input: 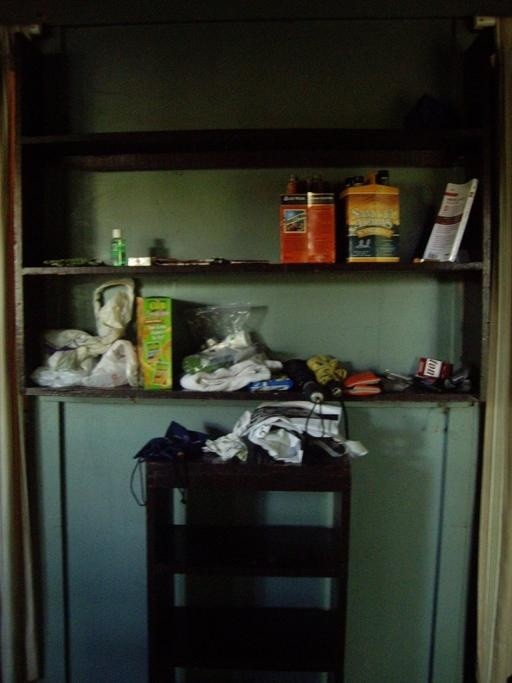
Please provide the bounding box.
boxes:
[147,463,351,683]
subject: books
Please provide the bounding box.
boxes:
[421,177,480,262]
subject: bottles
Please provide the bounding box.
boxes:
[111,228,126,267]
[345,177,352,187]
[352,176,364,186]
[378,170,389,185]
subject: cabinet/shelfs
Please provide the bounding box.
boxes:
[0,16,512,683]
